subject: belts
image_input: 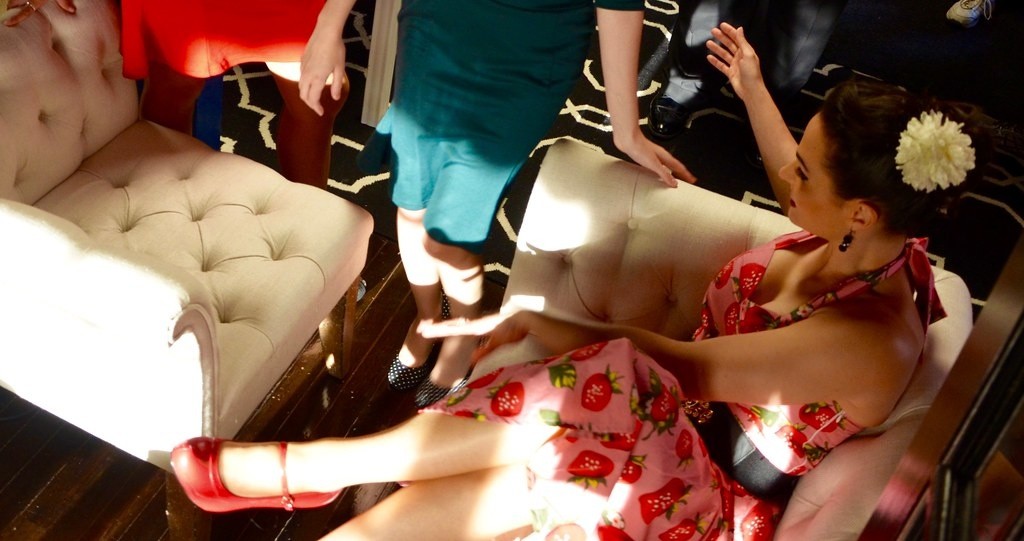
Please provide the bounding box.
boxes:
[681,401,801,509]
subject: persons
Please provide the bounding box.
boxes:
[297,0,697,405]
[170,22,976,541]
[2,0,76,26]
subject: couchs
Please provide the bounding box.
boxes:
[469,138,975,541]
[0,0,374,541]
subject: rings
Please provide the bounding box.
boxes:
[27,1,35,10]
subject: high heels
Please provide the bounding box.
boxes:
[386,295,454,391]
[414,318,484,409]
[175,439,342,514]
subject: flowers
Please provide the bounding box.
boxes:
[894,110,976,192]
